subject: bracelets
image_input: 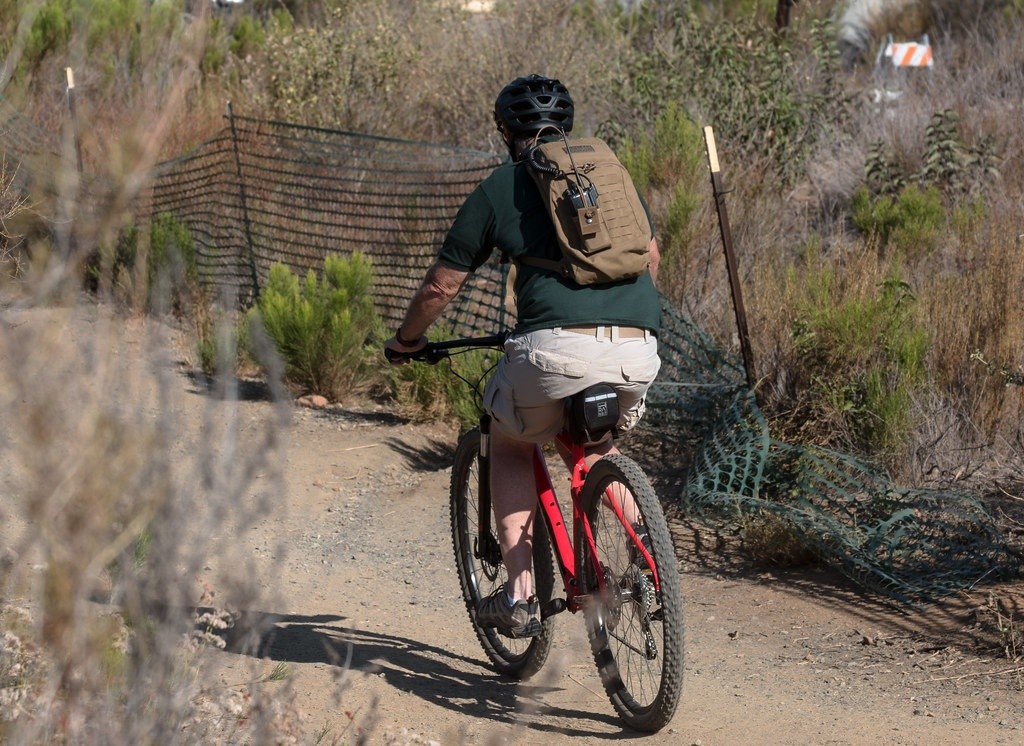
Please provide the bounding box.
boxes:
[396,327,422,347]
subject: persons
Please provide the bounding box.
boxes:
[381,74,681,639]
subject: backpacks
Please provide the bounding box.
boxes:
[515,136,651,285]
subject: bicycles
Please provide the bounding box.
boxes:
[384,335,686,734]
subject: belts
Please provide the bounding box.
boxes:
[561,325,655,338]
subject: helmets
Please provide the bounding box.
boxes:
[492,74,574,135]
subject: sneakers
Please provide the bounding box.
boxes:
[619,524,656,588]
[475,582,542,638]
